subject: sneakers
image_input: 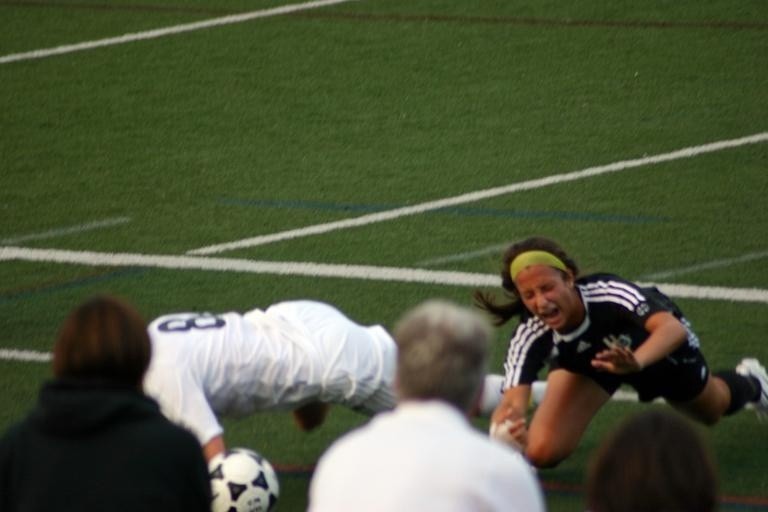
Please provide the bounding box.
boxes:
[734,358,768,423]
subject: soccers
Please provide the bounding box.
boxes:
[207,447,280,512]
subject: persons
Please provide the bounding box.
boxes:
[0,286,213,509]
[138,298,549,473]
[470,235,767,469]
[306,298,548,511]
[586,404,721,511]
